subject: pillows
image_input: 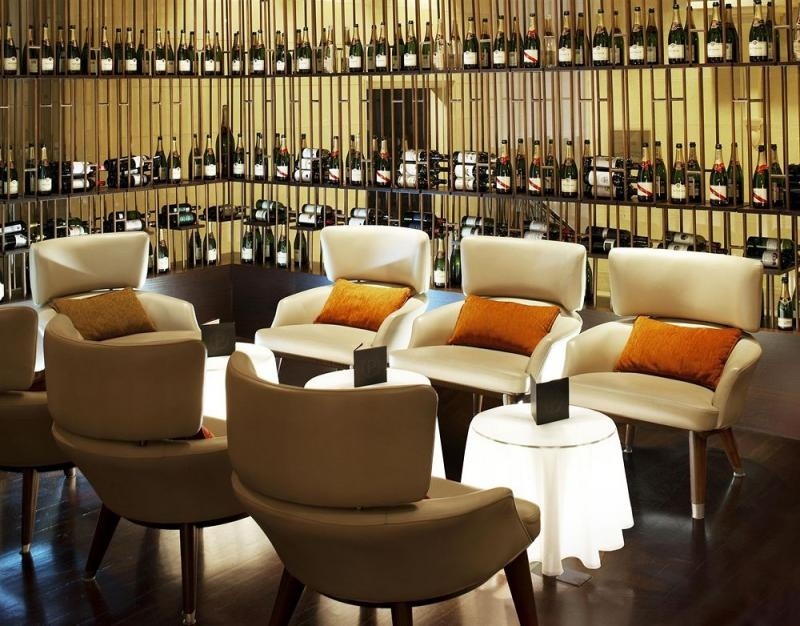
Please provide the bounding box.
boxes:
[312,278,415,333]
[446,295,562,359]
[612,315,742,392]
[48,285,155,342]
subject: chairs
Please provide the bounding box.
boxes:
[42,314,252,626]
[253,223,434,367]
[25,231,203,347]
[0,303,78,554]
[386,233,587,414]
[224,352,541,626]
[558,246,764,520]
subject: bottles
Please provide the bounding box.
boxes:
[2,0,799,338]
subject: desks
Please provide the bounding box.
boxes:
[197,335,279,420]
[300,367,445,480]
[458,396,631,585]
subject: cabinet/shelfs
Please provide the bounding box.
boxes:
[0,1,799,290]
